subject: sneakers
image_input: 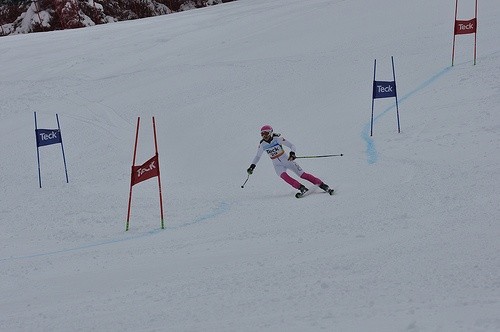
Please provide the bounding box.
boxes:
[322,184,335,196]
[295,185,309,198]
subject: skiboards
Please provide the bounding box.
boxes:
[295,186,334,199]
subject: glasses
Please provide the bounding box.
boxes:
[261,133,270,136]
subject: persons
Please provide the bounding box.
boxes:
[247,125,334,198]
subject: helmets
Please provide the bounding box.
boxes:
[260,124,274,132]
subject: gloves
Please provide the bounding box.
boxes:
[247,164,256,175]
[287,151,296,162]
